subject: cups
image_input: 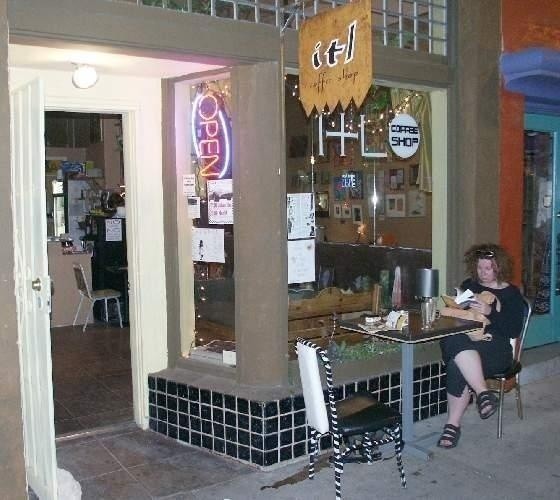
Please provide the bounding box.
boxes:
[420,303,434,328]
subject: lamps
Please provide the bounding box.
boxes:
[72,61,99,89]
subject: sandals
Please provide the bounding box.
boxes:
[437,423,462,449]
[475,391,499,420]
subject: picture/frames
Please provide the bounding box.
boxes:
[408,163,421,186]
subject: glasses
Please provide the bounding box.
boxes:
[474,250,494,257]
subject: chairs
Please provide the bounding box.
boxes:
[72,262,124,333]
[485,295,533,440]
[293,335,407,496]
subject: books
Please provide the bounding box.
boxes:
[359,311,409,335]
[442,283,480,310]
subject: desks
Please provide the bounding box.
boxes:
[339,310,484,444]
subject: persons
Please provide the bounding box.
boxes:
[438,244,523,449]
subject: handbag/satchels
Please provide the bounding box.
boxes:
[438,307,493,342]
